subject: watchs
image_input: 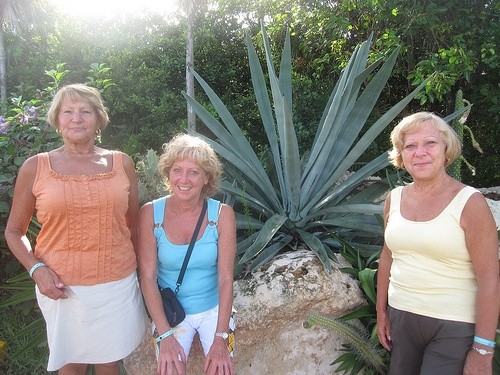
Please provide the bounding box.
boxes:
[215,331,229,339]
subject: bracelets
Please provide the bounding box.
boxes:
[474,336,496,347]
[156,330,173,342]
[29,262,46,278]
[472,345,495,355]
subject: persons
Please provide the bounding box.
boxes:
[376,112,500,375]
[5,82,148,375]
[137,132,240,375]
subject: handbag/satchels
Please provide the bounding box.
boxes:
[145,288,186,333]
